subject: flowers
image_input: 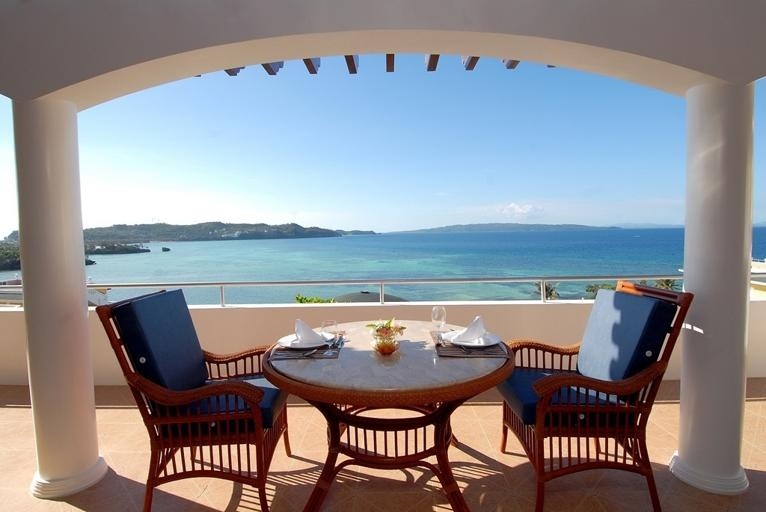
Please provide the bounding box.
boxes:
[364,317,407,338]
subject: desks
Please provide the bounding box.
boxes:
[261,319,515,511]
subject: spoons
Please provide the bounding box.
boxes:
[273,349,317,356]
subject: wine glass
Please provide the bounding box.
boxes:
[321,320,338,356]
[431,306,446,336]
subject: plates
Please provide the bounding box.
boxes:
[278,331,336,349]
[444,331,502,347]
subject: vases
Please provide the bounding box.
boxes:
[369,331,401,356]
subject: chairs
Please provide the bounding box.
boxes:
[95,288,292,512]
[494,280,695,511]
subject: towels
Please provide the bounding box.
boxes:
[451,316,486,342]
[294,318,322,343]
